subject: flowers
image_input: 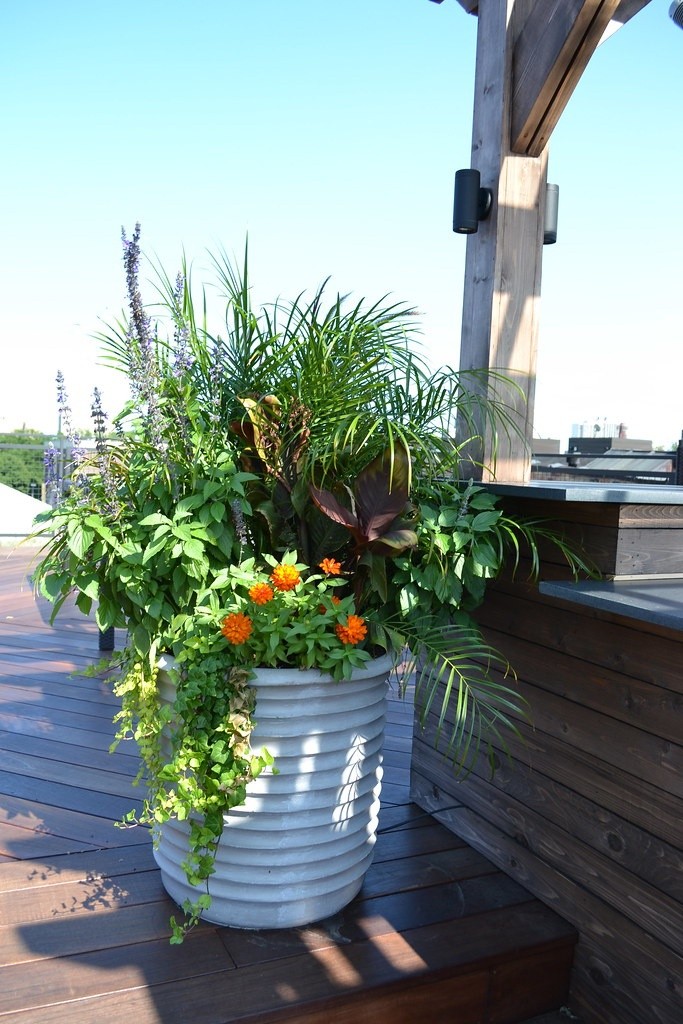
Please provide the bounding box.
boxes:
[39,214,256,547]
[217,550,372,681]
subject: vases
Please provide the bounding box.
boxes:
[136,641,406,929]
